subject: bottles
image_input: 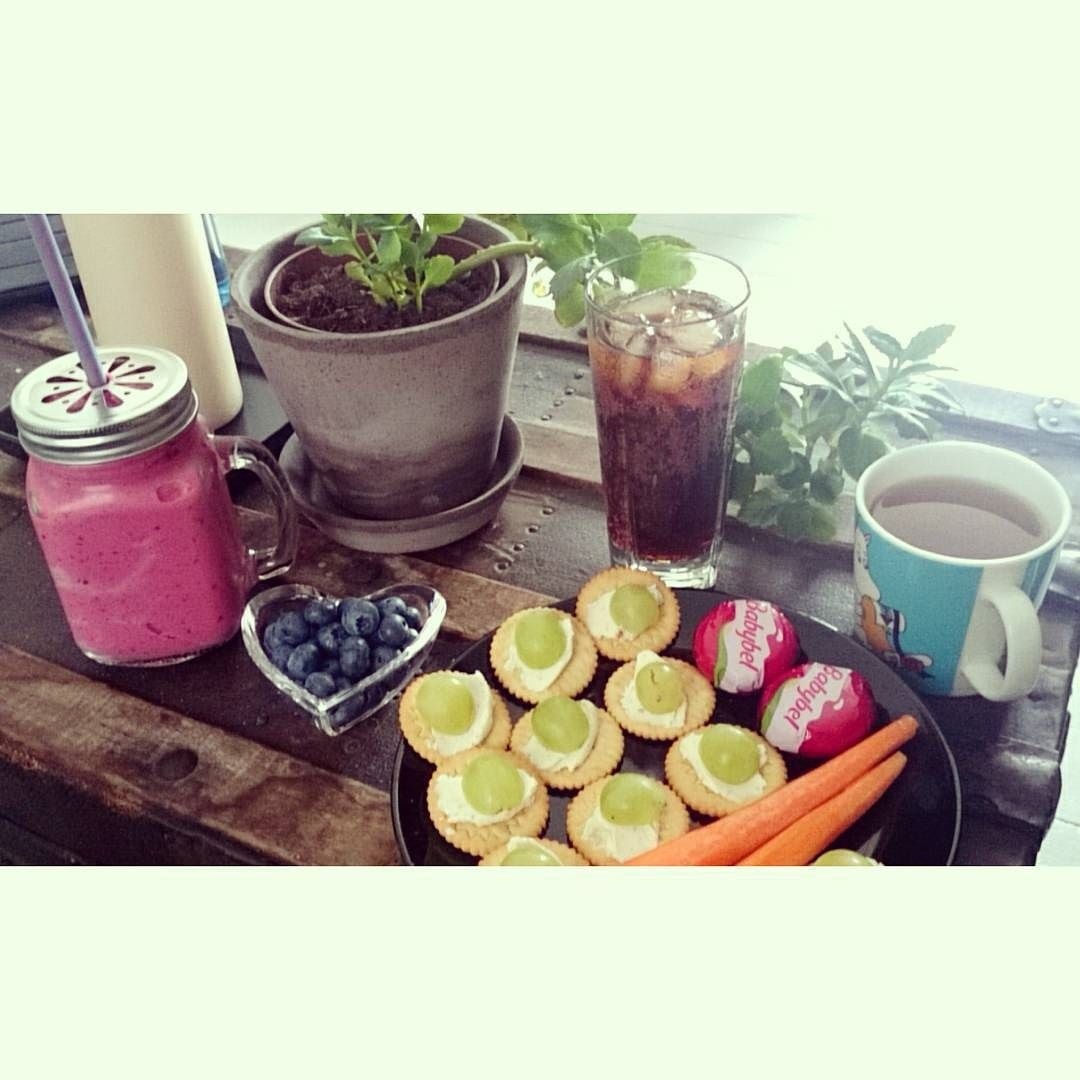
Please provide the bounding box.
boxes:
[63,213,242,433]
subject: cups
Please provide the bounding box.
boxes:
[10,347,299,668]
[582,248,751,589]
[851,439,1074,702]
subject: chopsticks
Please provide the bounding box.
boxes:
[617,713,920,866]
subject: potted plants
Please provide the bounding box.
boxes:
[229,213,966,552]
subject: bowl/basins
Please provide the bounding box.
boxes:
[239,581,446,738]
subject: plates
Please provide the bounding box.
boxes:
[390,589,960,867]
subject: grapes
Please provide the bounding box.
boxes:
[462,751,521,814]
[532,694,589,753]
[610,584,659,635]
[809,849,883,866]
[700,725,759,785]
[602,773,659,824]
[637,662,684,712]
[516,611,565,668]
[417,676,476,735]
[501,846,556,867]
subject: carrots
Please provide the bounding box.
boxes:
[618,715,919,866]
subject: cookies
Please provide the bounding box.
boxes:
[398,568,788,866]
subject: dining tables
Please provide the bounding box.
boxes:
[0,246,1080,866]
